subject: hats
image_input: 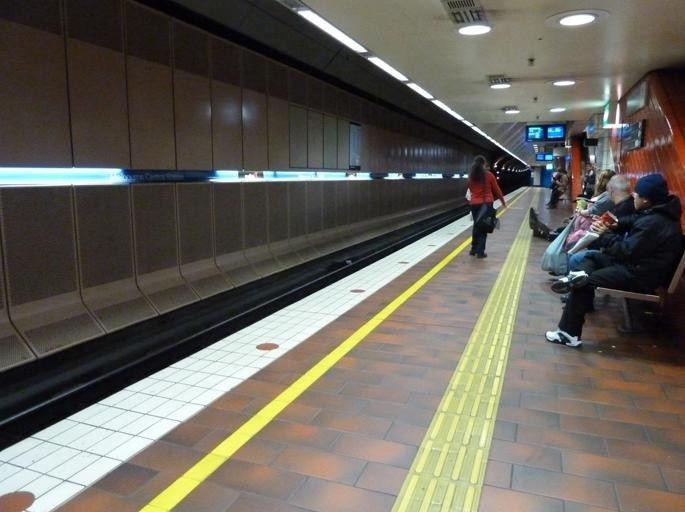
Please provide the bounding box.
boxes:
[634,173,669,205]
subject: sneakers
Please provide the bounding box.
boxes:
[544,329,583,348]
[550,267,590,293]
[469,246,487,258]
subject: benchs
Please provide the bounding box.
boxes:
[595,252,685,332]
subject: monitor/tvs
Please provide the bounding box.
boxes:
[526,124,545,142]
[545,124,566,141]
[545,154,553,161]
[536,154,545,161]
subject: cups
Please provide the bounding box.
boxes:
[590,211,618,234]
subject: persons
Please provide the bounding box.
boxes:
[465,154,508,259]
[529,161,684,349]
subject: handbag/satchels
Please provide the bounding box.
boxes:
[538,214,576,274]
[472,203,498,234]
